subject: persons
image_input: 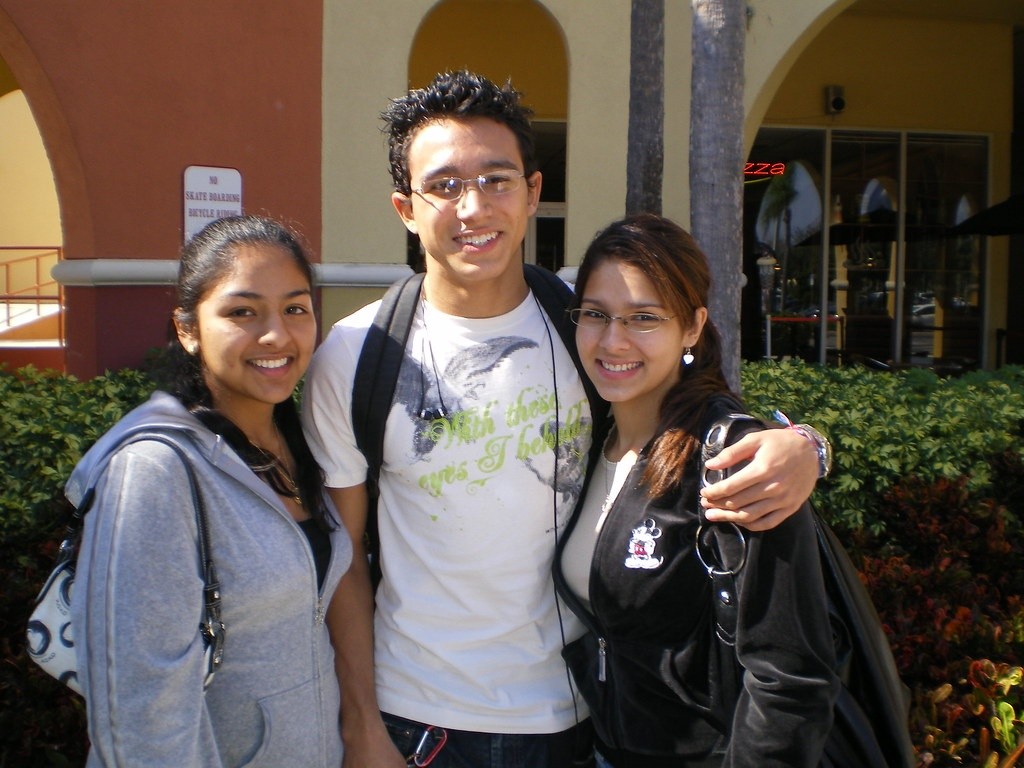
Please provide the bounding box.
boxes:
[553,214,861,768]
[304,70,831,768]
[65,217,352,768]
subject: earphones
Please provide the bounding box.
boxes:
[433,403,447,420]
[417,409,432,420]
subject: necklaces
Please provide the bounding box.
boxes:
[249,420,300,502]
[602,458,611,511]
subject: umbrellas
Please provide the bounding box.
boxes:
[794,205,952,267]
[946,195,1010,236]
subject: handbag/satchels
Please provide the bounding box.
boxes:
[23,438,226,700]
[709,412,915,732]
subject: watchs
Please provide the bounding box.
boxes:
[794,424,831,470]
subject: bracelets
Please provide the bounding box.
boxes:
[774,410,827,479]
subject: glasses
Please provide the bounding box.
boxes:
[562,307,684,334]
[409,171,532,204]
